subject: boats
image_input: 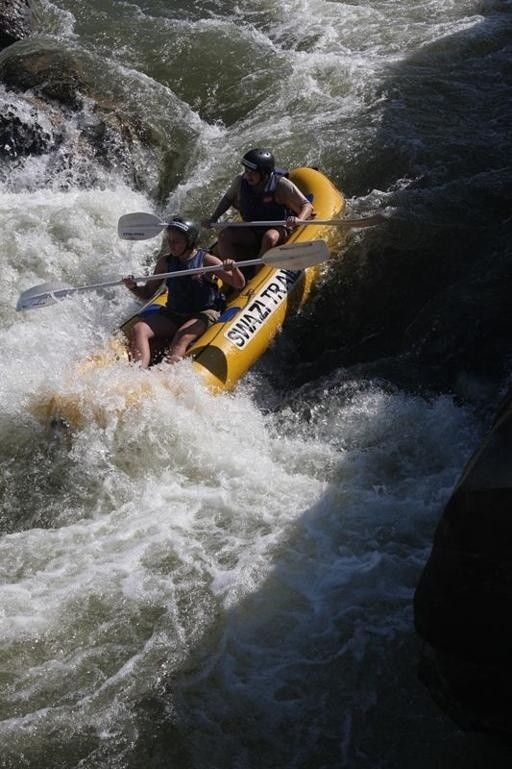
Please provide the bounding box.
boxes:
[28,159,345,451]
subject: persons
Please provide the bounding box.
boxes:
[122,216,246,371]
[202,149,313,276]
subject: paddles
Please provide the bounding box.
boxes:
[118,212,390,240]
[18,238,330,315]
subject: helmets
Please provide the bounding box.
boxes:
[166,218,199,250]
[241,149,274,173]
